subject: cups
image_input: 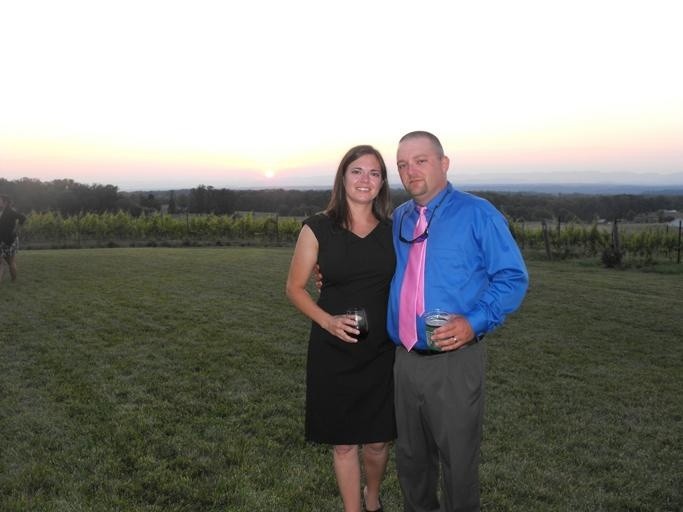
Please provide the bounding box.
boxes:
[345,305,369,342]
[420,309,453,352]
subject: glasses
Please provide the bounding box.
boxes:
[400,206,437,244]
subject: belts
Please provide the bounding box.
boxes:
[417,332,483,357]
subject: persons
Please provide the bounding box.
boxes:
[0,193,30,284]
[313,130,529,512]
[286,145,397,512]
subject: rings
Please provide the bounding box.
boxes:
[452,336,457,343]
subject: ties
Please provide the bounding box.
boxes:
[398,206,428,351]
